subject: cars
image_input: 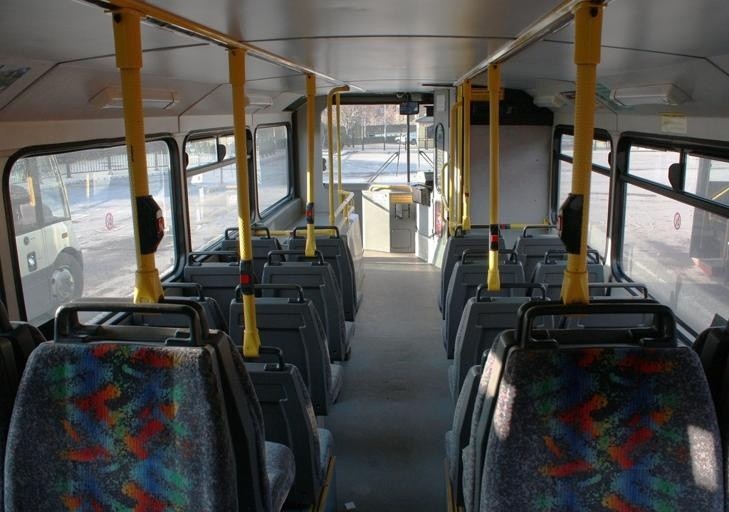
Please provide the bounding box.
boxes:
[396,132,417,144]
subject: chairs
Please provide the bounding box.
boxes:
[437,223,727,512]
[1,225,358,512]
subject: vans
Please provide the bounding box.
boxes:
[10,184,84,306]
